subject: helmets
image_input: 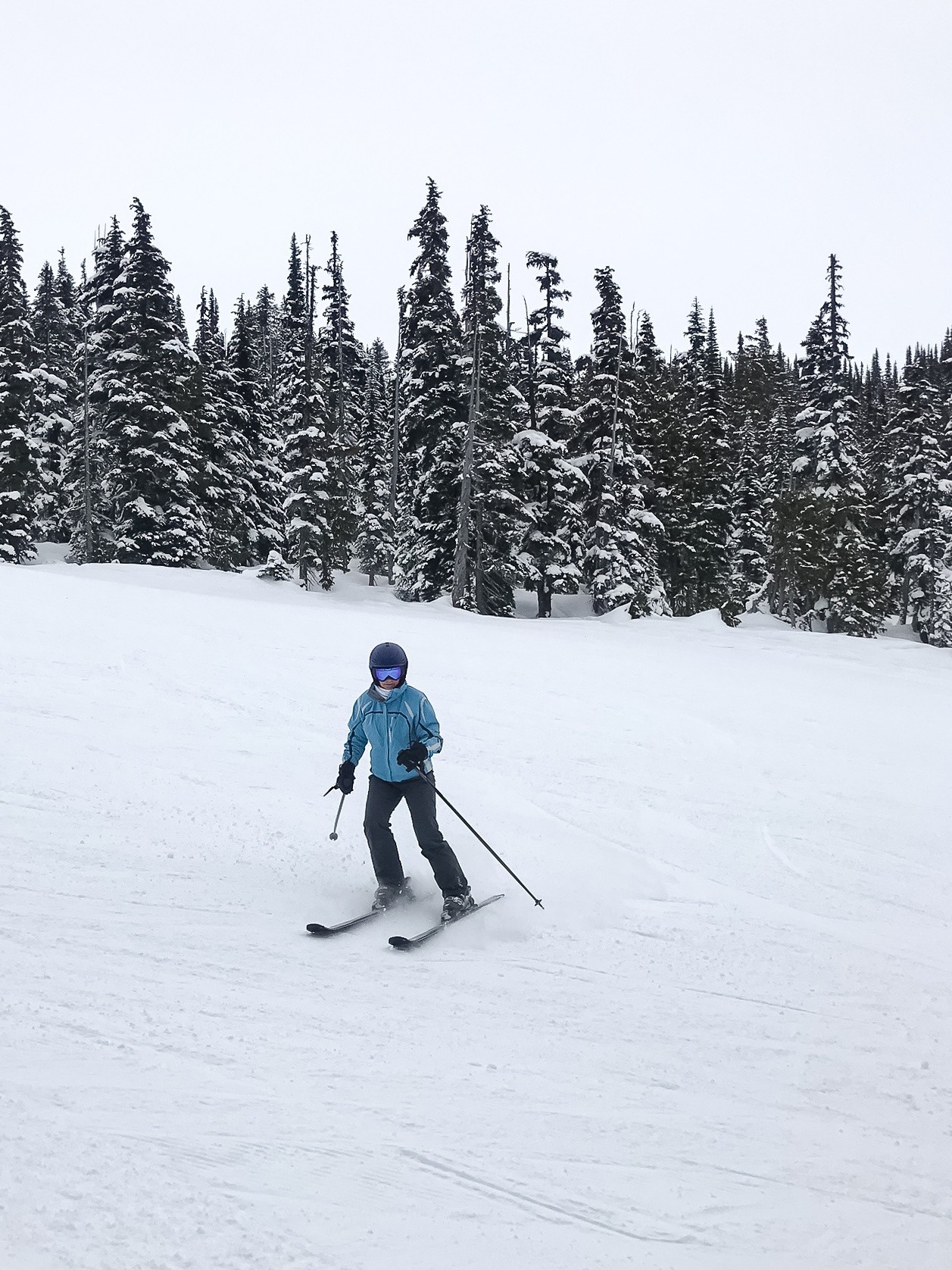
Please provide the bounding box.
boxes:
[369,642,408,690]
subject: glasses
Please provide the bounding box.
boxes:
[374,667,401,681]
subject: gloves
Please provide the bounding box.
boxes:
[337,762,356,795]
[397,742,429,773]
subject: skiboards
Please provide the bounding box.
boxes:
[305,893,504,949]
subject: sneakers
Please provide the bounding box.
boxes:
[441,884,474,922]
[374,879,405,903]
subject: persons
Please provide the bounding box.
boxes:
[336,643,474,921]
